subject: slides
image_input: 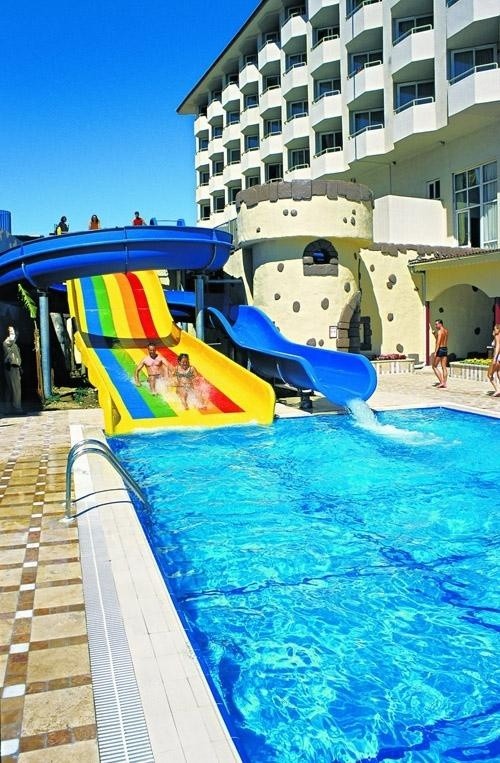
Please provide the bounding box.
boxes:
[0,224,377,410]
[67,267,277,439]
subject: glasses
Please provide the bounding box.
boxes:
[179,361,188,365]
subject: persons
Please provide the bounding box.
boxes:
[87,213,101,229]
[2,322,28,415]
[130,210,148,225]
[134,341,177,395]
[486,323,500,397]
[431,317,449,388]
[54,214,71,233]
[169,353,209,410]
